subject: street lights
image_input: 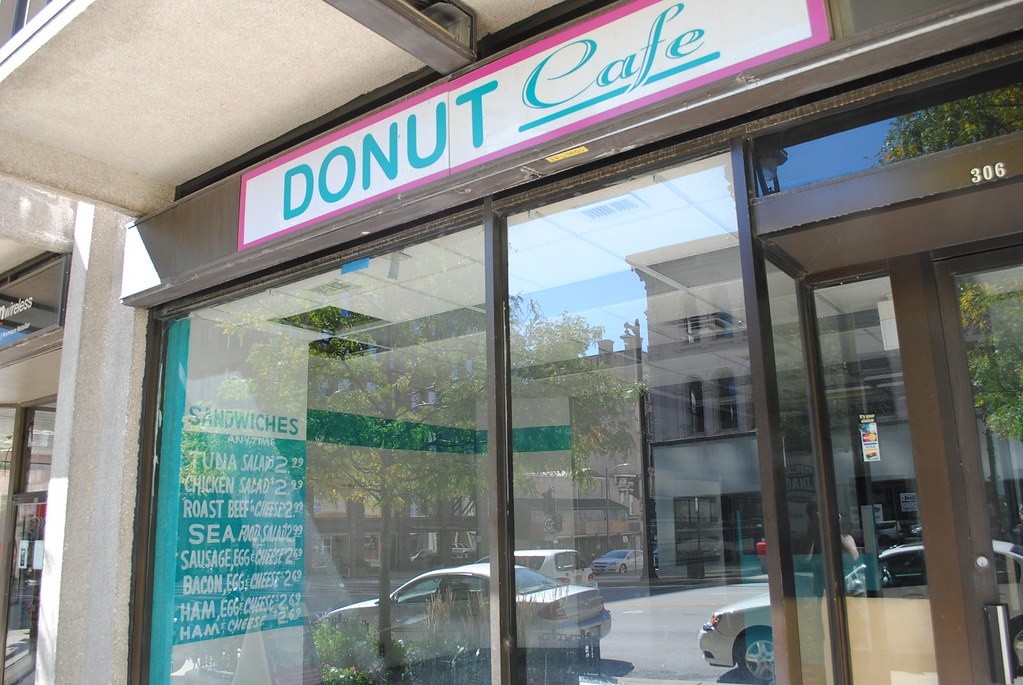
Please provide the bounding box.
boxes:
[620,320,659,579]
[604,463,631,552]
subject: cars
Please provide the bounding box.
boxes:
[589,549,655,575]
[853,522,904,544]
[696,540,1023,685]
[410,549,438,562]
[473,548,599,590]
[317,563,612,654]
[452,543,475,559]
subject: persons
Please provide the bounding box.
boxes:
[798,492,872,685]
